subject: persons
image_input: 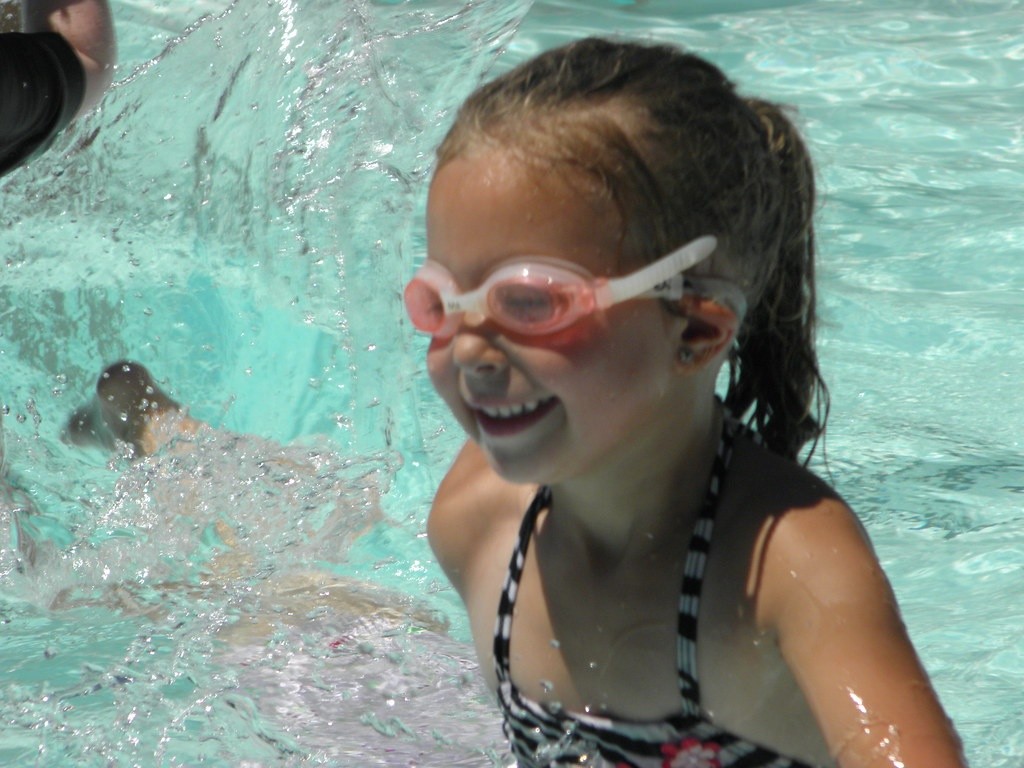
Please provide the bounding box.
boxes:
[400,39,965,768]
[0,0,117,180]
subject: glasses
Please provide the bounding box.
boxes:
[402,233,745,339]
